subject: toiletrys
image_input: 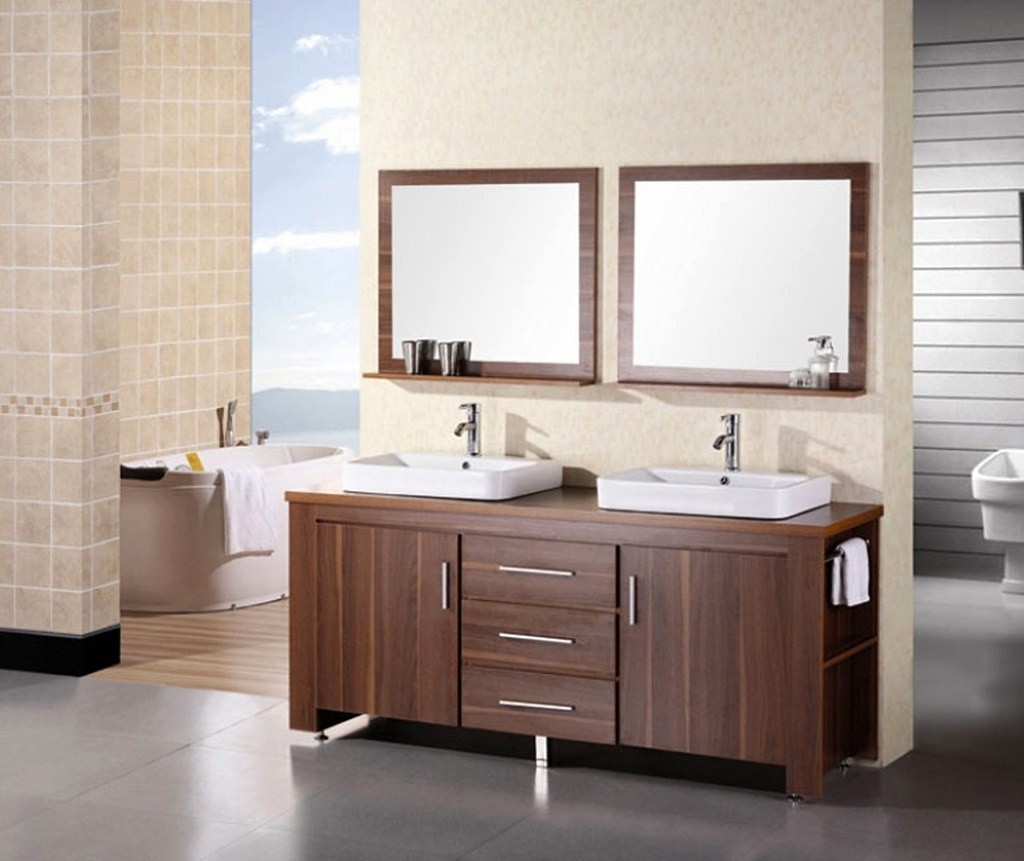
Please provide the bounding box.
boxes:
[826,335,839,373]
[786,336,831,389]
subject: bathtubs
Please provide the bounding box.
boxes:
[119,444,352,613]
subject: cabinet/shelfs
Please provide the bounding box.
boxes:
[283,488,885,801]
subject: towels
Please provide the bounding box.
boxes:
[831,538,870,607]
[215,466,276,556]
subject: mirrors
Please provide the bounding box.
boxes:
[618,163,868,398]
[363,167,600,387]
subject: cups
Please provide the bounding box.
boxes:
[438,341,472,376]
[402,339,437,375]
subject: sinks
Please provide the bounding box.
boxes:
[971,449,1024,594]
[596,468,832,520]
[342,453,563,500]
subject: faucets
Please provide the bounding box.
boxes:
[712,414,741,474]
[454,403,482,457]
[224,398,239,448]
